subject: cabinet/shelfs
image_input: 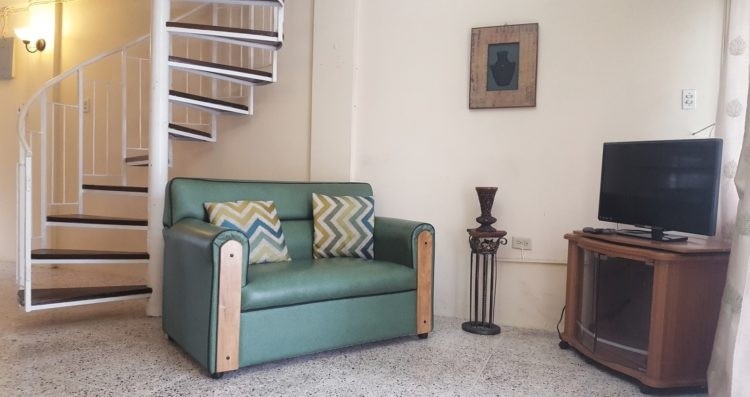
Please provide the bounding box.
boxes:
[558,228,732,393]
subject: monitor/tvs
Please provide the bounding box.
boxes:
[598,137,723,243]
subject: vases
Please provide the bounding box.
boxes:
[475,187,498,231]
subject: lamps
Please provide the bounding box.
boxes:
[14,29,46,53]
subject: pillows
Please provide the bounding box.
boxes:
[203,199,292,265]
[311,193,375,259]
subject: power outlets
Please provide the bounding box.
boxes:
[682,90,697,108]
[512,236,532,250]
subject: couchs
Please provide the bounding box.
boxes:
[161,177,435,380]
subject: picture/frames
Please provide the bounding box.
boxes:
[469,22,539,108]
